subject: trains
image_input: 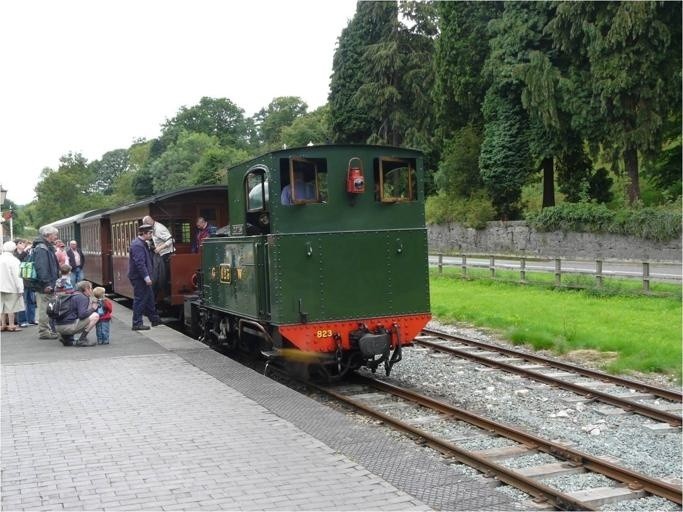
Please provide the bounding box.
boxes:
[37,144,432,385]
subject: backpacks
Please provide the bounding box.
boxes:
[46,293,80,320]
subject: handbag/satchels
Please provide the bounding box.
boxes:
[20,262,37,281]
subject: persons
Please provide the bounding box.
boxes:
[140,216,177,303]
[0,227,112,345]
[124,225,167,331]
[190,218,218,254]
[281,169,320,206]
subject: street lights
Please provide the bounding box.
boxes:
[0,185,8,211]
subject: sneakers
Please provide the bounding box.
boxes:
[20,320,39,327]
[39,329,96,346]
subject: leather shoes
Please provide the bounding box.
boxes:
[132,325,150,330]
[152,320,169,327]
[1,326,22,332]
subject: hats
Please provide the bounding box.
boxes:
[139,225,154,231]
